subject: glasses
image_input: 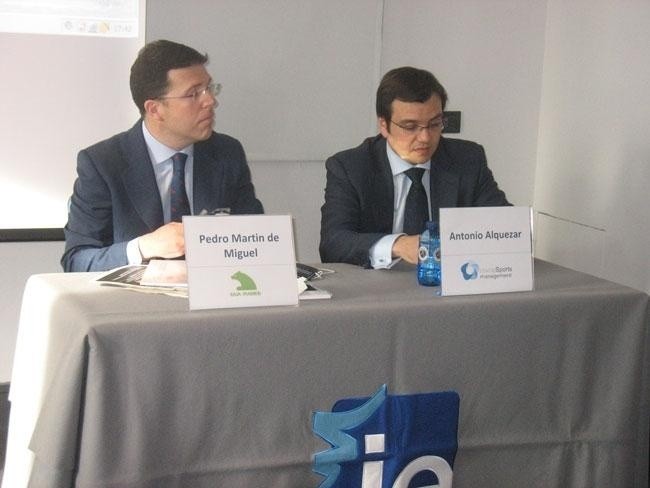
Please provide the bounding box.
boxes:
[391,119,444,133]
[159,84,220,101]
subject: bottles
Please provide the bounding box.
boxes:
[418,222,440,286]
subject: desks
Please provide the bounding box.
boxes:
[1,256,649,488]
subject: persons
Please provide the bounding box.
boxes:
[318,66,516,270]
[59,40,265,273]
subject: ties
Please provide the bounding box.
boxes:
[404,168,429,235]
[170,153,191,222]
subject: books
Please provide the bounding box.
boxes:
[140,258,189,288]
